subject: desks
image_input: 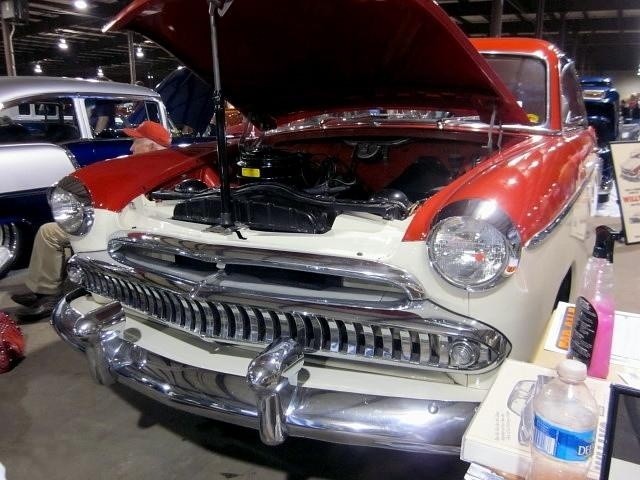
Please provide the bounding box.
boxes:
[467,301,640,478]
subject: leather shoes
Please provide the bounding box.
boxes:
[11,292,51,307]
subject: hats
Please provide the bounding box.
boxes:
[123,120,170,147]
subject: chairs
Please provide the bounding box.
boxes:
[2,118,78,145]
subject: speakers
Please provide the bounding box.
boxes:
[1,0,30,22]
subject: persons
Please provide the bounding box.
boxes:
[619,94,639,120]
[10,120,174,322]
[88,76,116,134]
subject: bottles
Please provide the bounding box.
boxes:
[524,358,600,480]
